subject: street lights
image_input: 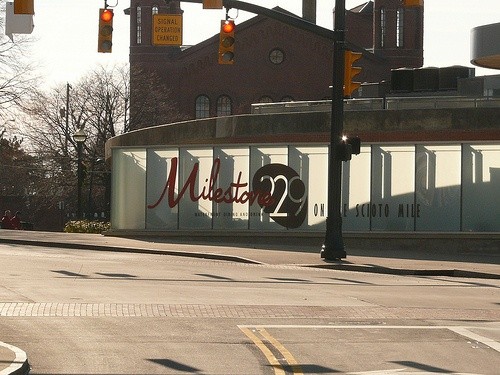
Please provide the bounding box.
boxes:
[73,130,87,218]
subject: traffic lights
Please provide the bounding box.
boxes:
[219,20,236,65]
[344,50,362,96]
[339,135,360,162]
[98,9,114,53]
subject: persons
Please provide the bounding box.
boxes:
[9,211,24,230]
[1,210,17,230]
